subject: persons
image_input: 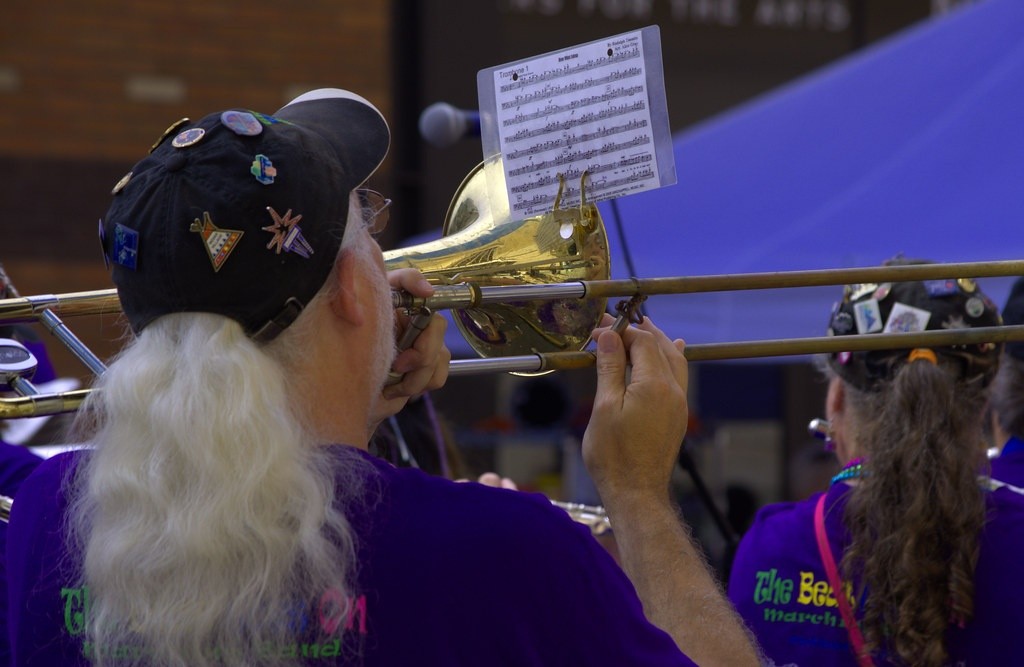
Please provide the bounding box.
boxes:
[981,360,1022,489]
[0,325,79,526]
[0,89,763,667]
[728,260,1023,667]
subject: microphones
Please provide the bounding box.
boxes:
[418,102,481,147]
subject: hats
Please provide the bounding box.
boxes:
[97,87,392,352]
[825,258,1003,405]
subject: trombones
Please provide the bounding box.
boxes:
[352,257,1024,395]
[0,148,613,420]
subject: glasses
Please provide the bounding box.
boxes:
[352,187,391,236]
